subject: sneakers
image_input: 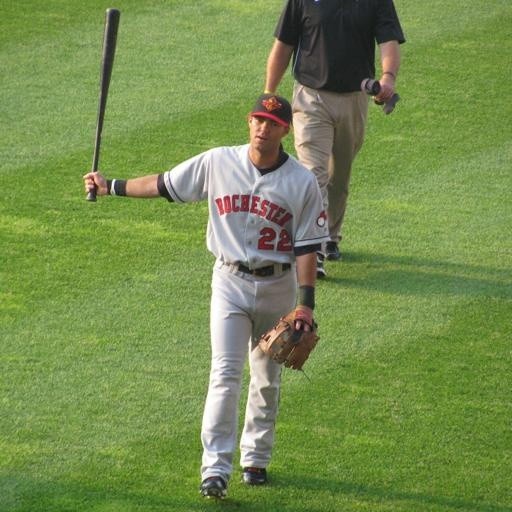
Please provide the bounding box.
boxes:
[325,242,340,259]
[316,253,326,277]
[199,476,228,500]
[242,467,267,485]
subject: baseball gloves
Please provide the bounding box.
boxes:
[257,311,320,371]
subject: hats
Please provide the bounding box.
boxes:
[250,94,293,128]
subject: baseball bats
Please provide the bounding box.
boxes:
[86,8,121,201]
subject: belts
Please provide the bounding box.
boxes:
[224,260,291,277]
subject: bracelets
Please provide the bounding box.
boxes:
[107,177,127,198]
[264,89,273,95]
[384,71,396,80]
[298,283,315,311]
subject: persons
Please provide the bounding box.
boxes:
[83,92,334,501]
[262,1,405,280]
